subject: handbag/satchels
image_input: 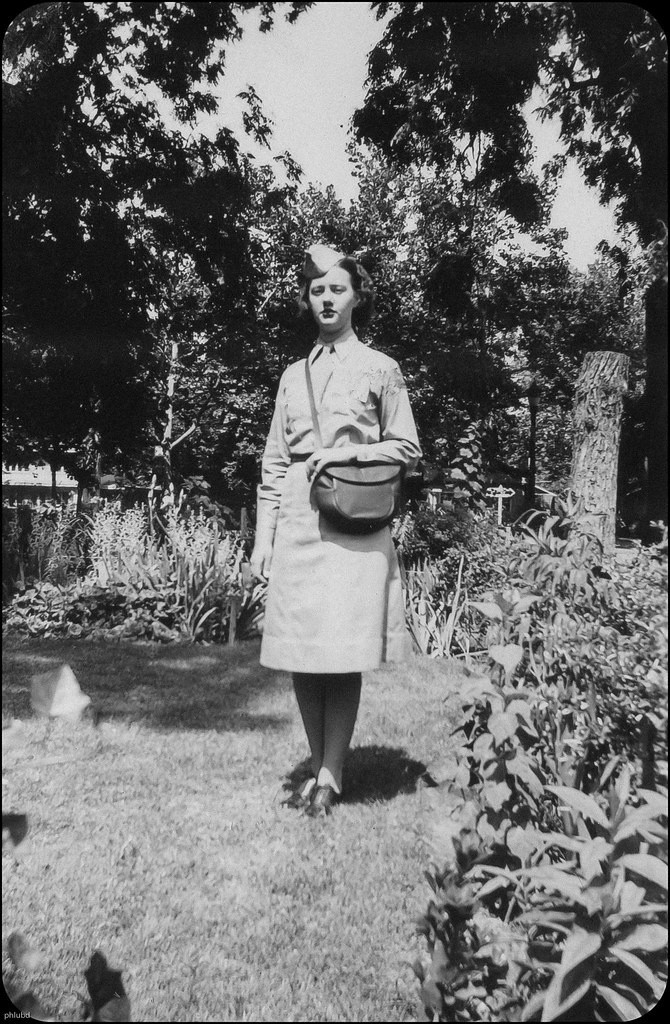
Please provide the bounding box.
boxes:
[310,454,407,530]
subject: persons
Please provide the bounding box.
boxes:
[251,245,423,816]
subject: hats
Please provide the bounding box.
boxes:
[304,244,345,278]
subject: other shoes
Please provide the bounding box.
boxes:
[282,773,344,818]
